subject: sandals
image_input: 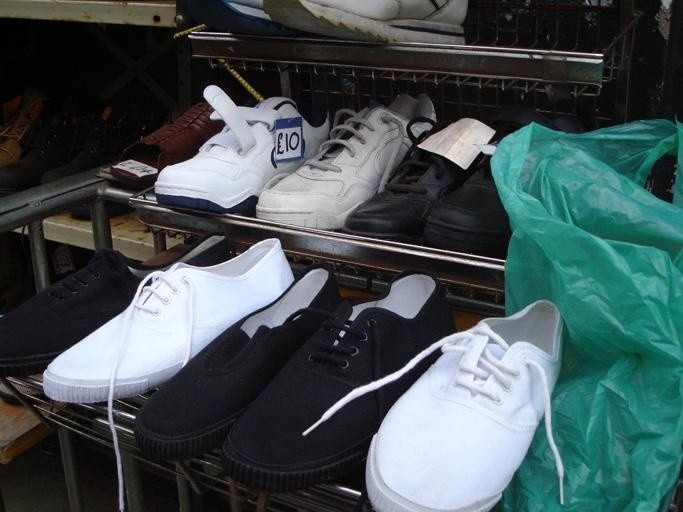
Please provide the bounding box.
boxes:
[114,99,227,184]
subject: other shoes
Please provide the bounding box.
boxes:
[2,89,55,169]
[2,105,86,193]
[72,199,134,220]
[43,104,148,189]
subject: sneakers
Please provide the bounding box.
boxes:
[423,107,557,251]
[41,236,297,511]
[343,118,499,231]
[254,92,437,229]
[1,244,209,380]
[152,85,334,212]
[175,0,468,47]
[133,267,343,461]
[298,300,567,510]
[220,270,458,491]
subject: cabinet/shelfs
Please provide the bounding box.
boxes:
[0,0,638,510]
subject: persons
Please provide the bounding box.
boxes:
[631,0,666,119]
